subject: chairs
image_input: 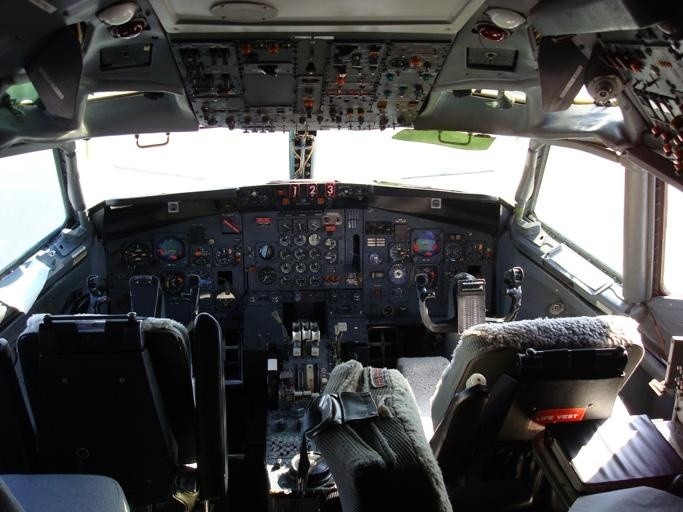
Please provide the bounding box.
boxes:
[312,361,456,511]
[18,313,229,505]
[395,314,646,482]
